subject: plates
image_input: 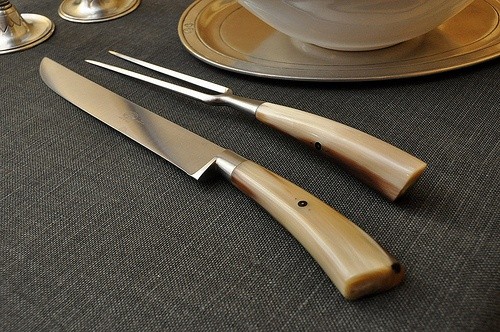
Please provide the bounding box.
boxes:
[178,0,500,80]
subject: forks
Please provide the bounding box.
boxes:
[86,51,427,200]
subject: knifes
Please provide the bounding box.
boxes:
[39,57,402,298]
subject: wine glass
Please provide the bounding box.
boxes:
[58,0,140,22]
[0,0,54,54]
